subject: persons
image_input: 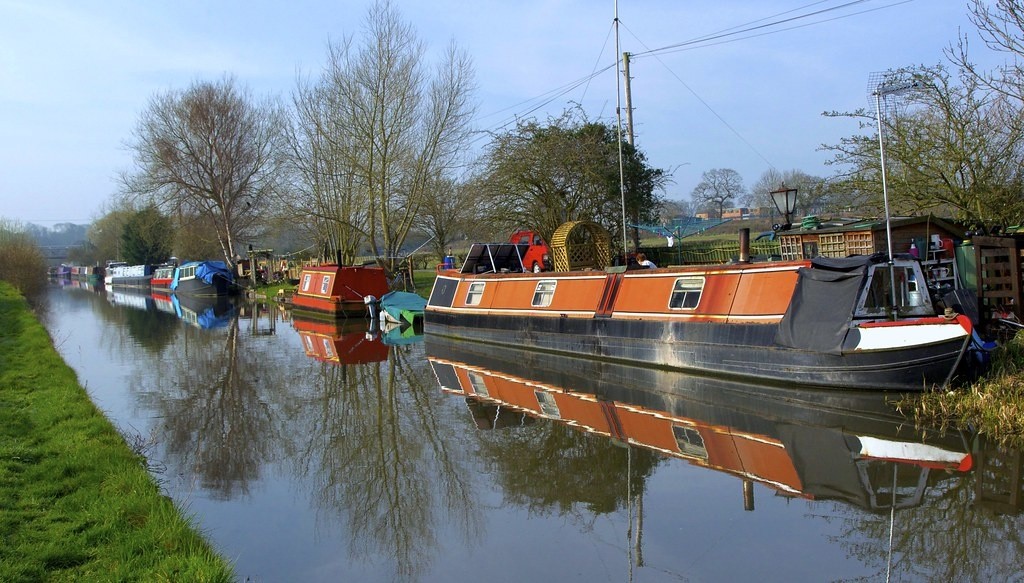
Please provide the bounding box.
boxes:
[636,253,657,269]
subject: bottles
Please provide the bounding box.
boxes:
[909,244,919,257]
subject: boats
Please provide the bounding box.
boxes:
[290,311,389,366]
[104,261,152,286]
[150,289,236,331]
[420,333,990,514]
[290,263,389,317]
[362,290,430,323]
[363,319,424,355]
[419,229,998,394]
[150,256,234,296]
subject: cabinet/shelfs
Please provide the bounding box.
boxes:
[921,257,959,298]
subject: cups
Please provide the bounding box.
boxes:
[930,234,943,250]
[941,268,949,278]
